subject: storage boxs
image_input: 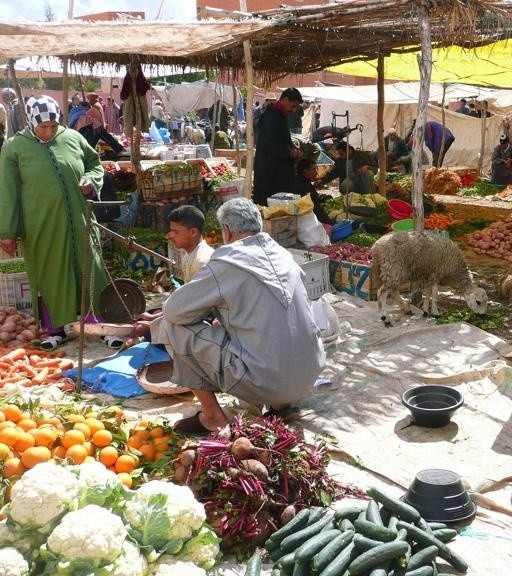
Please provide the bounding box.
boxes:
[330,259,411,302]
[139,163,203,201]
[127,241,166,274]
[263,216,297,247]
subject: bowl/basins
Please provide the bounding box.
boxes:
[387,198,413,220]
[331,218,355,242]
[398,468,476,521]
[100,279,145,323]
[391,217,414,232]
[401,385,466,428]
[481,183,505,192]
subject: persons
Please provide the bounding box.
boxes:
[455,97,491,118]
[1,88,16,144]
[294,159,337,225]
[252,92,276,130]
[316,143,378,193]
[407,119,455,167]
[490,134,512,186]
[127,204,215,352]
[149,196,328,438]
[372,128,408,166]
[0,94,125,351]
[288,104,304,135]
[313,126,351,143]
[68,95,125,151]
[252,88,301,207]
[92,172,121,223]
[208,97,231,149]
[149,100,166,128]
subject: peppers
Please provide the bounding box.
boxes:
[320,196,344,214]
[211,173,239,185]
[0,260,26,273]
[459,182,499,196]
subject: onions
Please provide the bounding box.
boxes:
[310,244,372,265]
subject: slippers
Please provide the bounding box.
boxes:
[176,410,213,435]
[262,406,299,420]
[40,335,69,351]
[99,335,124,349]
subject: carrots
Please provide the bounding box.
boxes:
[422,212,463,230]
[1,342,75,382]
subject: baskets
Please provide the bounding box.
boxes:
[0,258,33,312]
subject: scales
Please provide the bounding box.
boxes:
[76,193,176,393]
[310,110,379,220]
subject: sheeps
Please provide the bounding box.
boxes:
[368,229,490,328]
[183,125,206,145]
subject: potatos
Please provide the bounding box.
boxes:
[0,306,49,343]
[203,230,222,243]
[465,222,512,262]
[500,185,512,197]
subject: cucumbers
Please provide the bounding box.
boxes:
[244,488,468,576]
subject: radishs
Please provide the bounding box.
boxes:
[0,383,71,408]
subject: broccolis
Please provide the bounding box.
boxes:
[344,192,387,207]
[1,460,223,576]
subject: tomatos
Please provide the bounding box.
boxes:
[106,164,116,174]
[200,165,229,178]
